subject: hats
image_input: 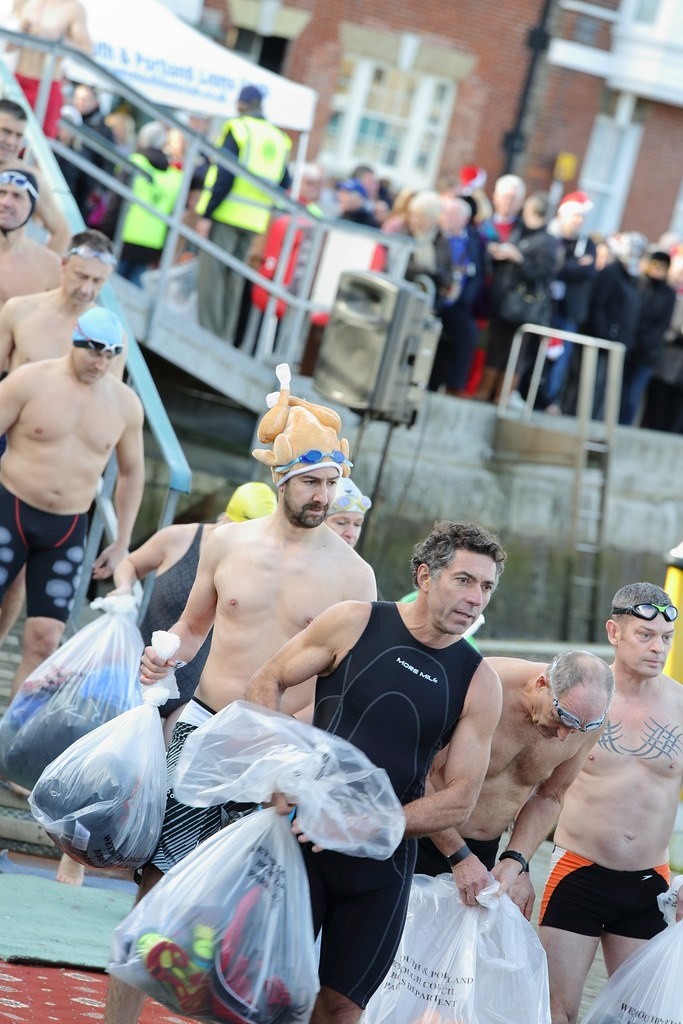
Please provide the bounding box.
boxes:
[251,362,354,484]
[0,168,38,199]
[556,192,594,213]
[240,86,261,105]
[326,476,366,516]
[72,307,124,345]
[225,481,278,522]
[458,165,486,194]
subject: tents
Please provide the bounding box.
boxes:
[0,0,320,212]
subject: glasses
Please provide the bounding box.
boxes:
[73,340,123,355]
[0,174,30,188]
[65,244,119,266]
[548,667,608,734]
[329,496,373,512]
[275,448,353,474]
[611,604,680,623]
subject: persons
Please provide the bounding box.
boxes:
[1,79,683,1024]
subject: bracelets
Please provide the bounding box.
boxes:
[447,844,470,868]
[499,850,526,875]
